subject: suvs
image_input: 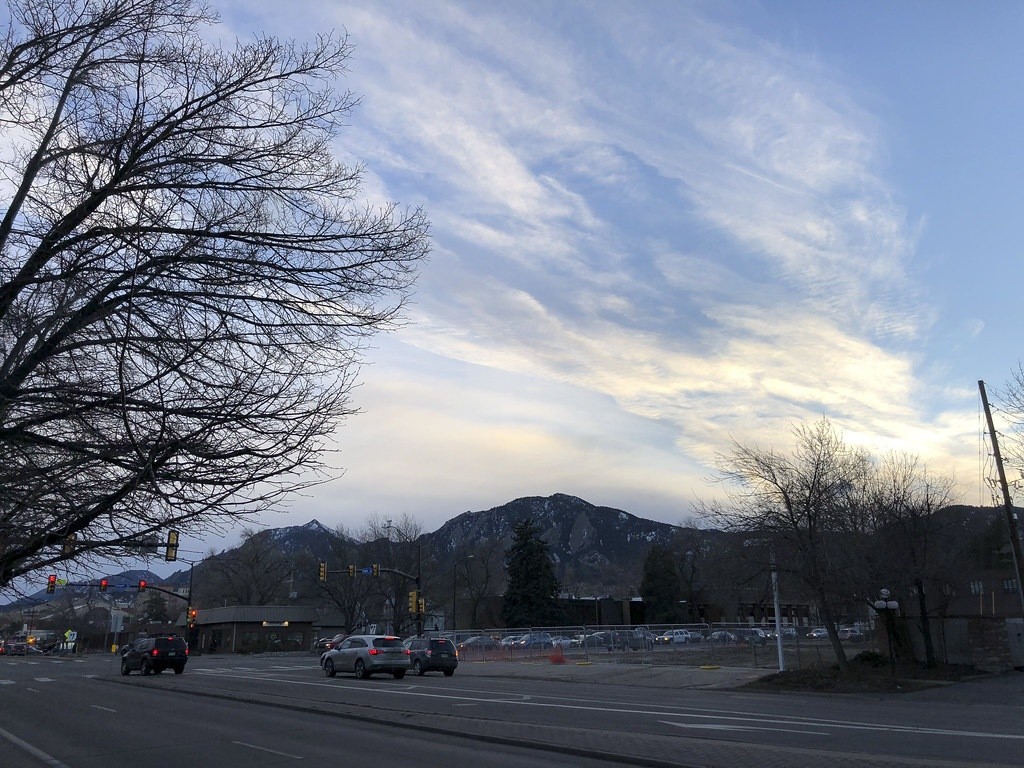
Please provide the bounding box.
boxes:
[119,635,189,678]
[402,634,458,676]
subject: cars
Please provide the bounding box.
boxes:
[314,633,351,656]
[318,634,411,679]
[121,638,148,657]
[0,631,69,660]
[401,594,865,653]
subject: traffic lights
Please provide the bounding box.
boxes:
[184,606,191,622]
[370,563,377,577]
[48,575,57,594]
[407,591,417,614]
[138,579,146,592]
[417,598,425,613]
[99,579,108,592]
[318,561,328,584]
[191,609,197,624]
[348,564,355,578]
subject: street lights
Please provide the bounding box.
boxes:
[744,537,785,673]
[871,588,904,688]
[452,556,476,632]
[381,519,422,593]
